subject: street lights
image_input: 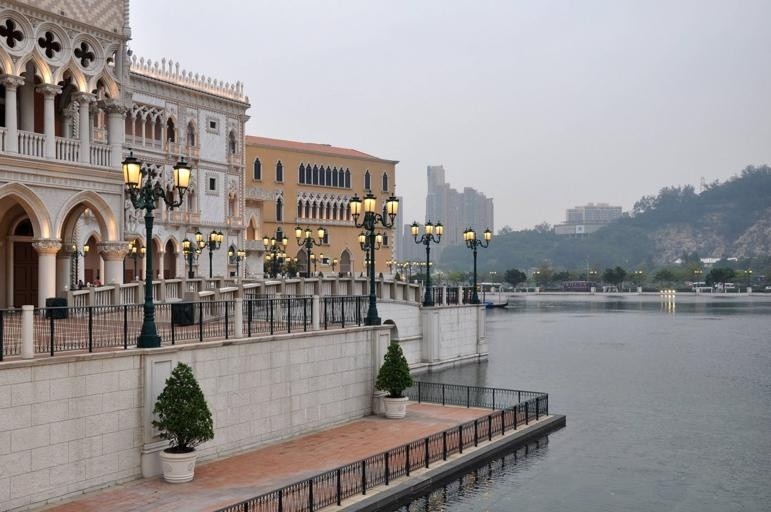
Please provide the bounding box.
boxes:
[181,230,205,279]
[532,270,541,288]
[634,269,643,288]
[358,230,383,296]
[198,227,224,279]
[262,233,298,279]
[325,257,338,272]
[692,269,702,284]
[589,271,597,288]
[385,259,432,283]
[119,148,190,345]
[345,189,398,329]
[71,243,89,290]
[128,239,146,283]
[464,227,491,301]
[410,220,439,308]
[294,223,325,278]
[744,270,753,286]
[228,248,247,276]
[309,252,324,277]
[489,271,496,283]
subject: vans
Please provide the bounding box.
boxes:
[717,282,736,290]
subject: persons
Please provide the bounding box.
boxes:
[72,276,101,289]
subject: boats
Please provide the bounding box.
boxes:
[467,282,509,308]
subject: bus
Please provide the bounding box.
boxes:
[561,280,591,291]
[691,281,717,290]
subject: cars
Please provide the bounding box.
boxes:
[714,284,717,288]
